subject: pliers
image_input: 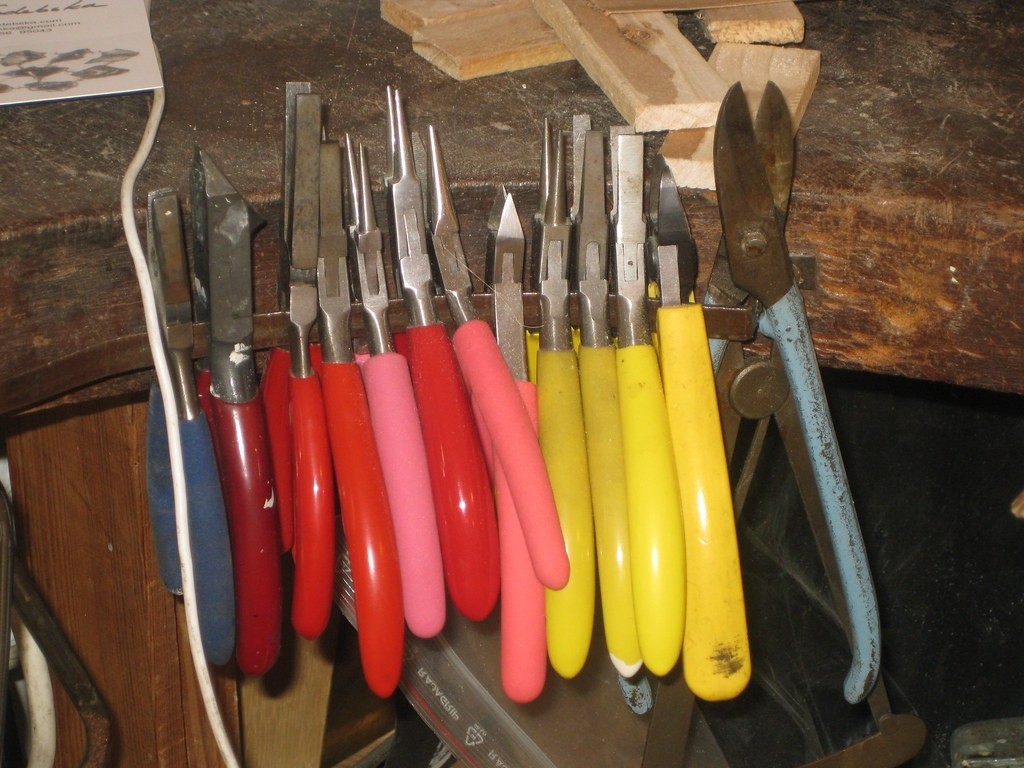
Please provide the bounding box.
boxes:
[146,79,883,717]
